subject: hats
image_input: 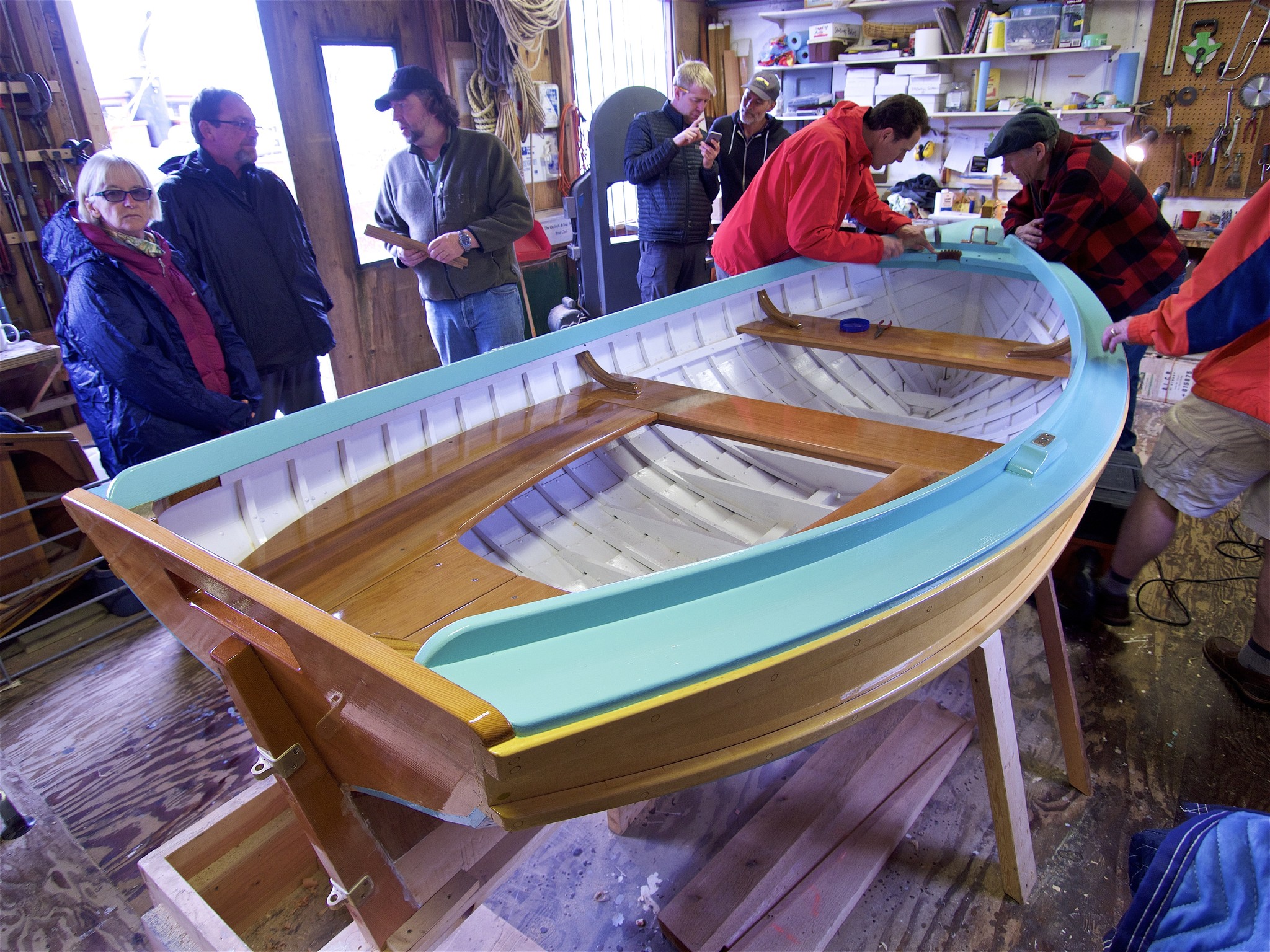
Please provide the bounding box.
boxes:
[90,188,152,202]
[740,71,781,101]
[984,107,1060,159]
[373,65,444,112]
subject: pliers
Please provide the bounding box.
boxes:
[1242,108,1257,143]
[874,320,892,339]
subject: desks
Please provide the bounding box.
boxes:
[0,333,76,429]
[1170,222,1227,248]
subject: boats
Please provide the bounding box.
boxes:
[58,213,1133,838]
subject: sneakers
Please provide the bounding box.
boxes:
[1203,634,1269,706]
[1063,577,1134,627]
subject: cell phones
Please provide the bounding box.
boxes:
[705,131,722,149]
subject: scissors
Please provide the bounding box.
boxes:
[1186,150,1202,192]
[1159,91,1177,129]
[1210,125,1231,166]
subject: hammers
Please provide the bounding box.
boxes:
[1164,124,1193,197]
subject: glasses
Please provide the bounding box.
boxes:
[206,120,263,132]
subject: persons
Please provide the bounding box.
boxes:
[623,61,720,304]
[709,92,936,279]
[1051,179,1269,713]
[374,65,535,364]
[983,106,1189,453]
[145,86,335,426]
[40,148,265,483]
[703,70,792,220]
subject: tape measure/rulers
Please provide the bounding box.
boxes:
[1162,0,1187,75]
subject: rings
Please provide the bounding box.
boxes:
[1111,328,1117,336]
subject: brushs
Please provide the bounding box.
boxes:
[1224,154,1243,188]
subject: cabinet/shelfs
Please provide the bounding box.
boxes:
[759,1,1128,231]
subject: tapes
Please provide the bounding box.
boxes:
[1081,33,1107,49]
[1096,94,1117,106]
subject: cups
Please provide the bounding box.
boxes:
[0,320,20,352]
[1181,209,1202,229]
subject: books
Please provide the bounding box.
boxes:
[933,2,994,55]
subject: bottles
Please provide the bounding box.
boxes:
[933,188,954,214]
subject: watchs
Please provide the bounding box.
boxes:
[457,230,471,252]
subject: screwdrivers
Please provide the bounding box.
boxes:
[44,199,56,219]
[13,179,28,217]
[1260,143,1270,185]
[29,182,49,220]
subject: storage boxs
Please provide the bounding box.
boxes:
[969,65,1004,115]
[1059,1,1087,49]
[1052,447,1146,595]
[805,21,863,46]
[1006,13,1061,55]
[806,41,855,64]
[1010,3,1063,19]
